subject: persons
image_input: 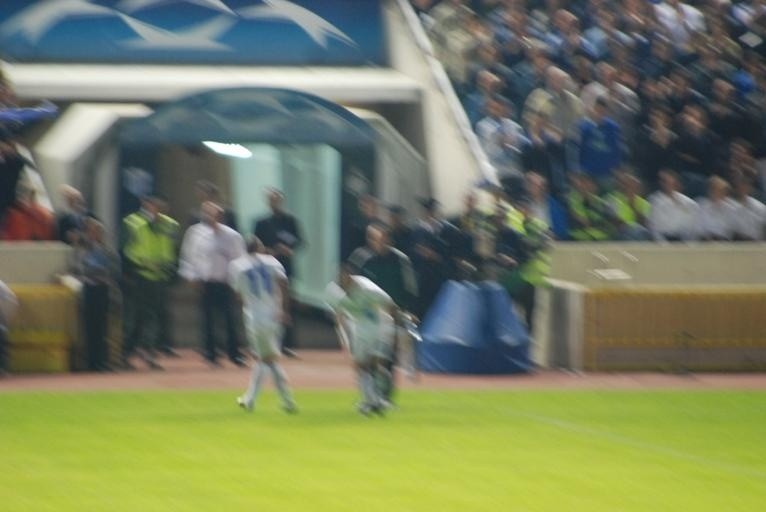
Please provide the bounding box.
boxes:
[228,234,300,413]
[0,59,411,417]
[409,0,766,242]
[53,188,302,369]
[0,74,59,242]
[323,221,411,415]
[349,193,553,334]
